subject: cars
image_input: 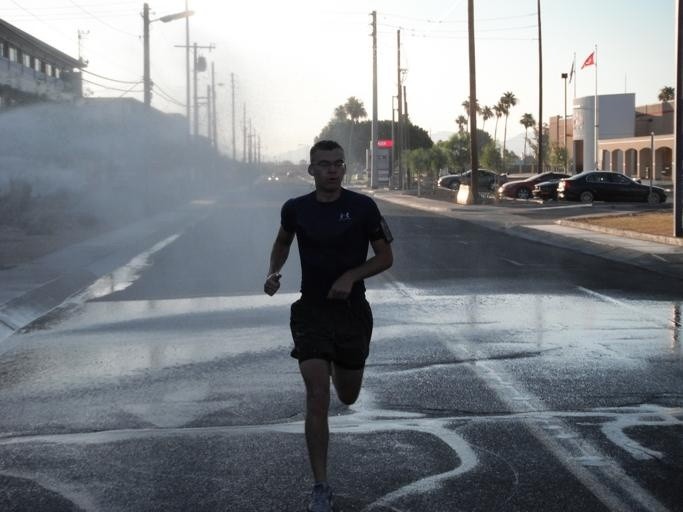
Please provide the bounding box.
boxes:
[286,171,297,178]
[531,178,564,199]
[558,170,667,205]
[413,176,433,186]
[499,171,570,200]
[436,169,505,191]
[267,173,281,183]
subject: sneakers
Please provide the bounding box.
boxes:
[308,480,335,512]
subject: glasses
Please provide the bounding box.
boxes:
[313,160,346,170]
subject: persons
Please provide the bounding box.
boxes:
[263,140,393,511]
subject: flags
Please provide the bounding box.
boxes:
[581,49,596,70]
[568,56,576,84]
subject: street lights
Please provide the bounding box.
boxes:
[649,128,656,187]
[297,142,308,164]
[561,72,568,174]
[139,1,197,109]
[211,81,225,150]
